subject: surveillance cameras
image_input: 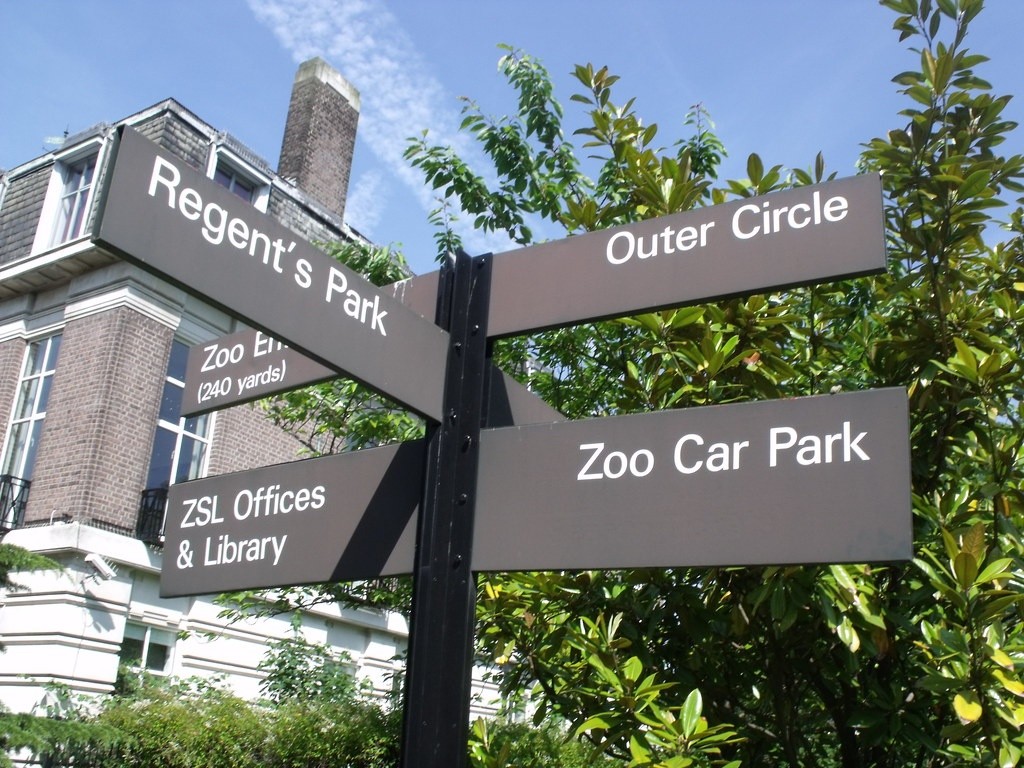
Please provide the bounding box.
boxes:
[85,553,117,581]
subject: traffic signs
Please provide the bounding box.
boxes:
[92,120,912,601]
